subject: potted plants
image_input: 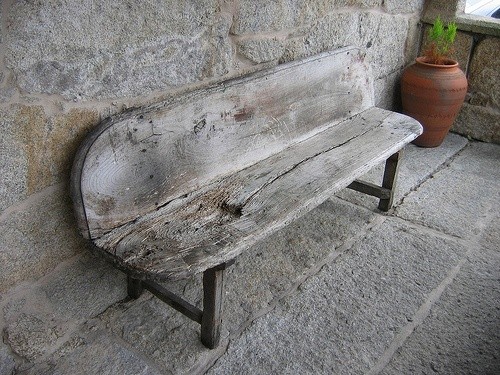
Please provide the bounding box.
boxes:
[400,14,468,149]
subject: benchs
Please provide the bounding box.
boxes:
[68,46,424,350]
